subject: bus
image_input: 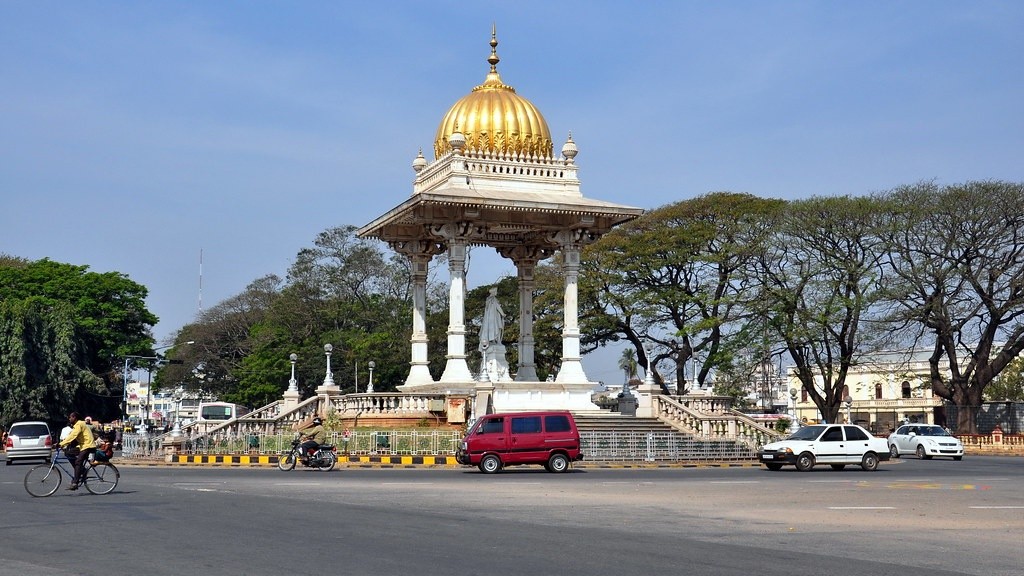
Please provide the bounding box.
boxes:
[195,400,249,438]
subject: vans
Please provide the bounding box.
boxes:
[455,409,585,475]
[3,420,53,466]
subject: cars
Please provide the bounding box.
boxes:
[755,422,893,473]
[887,422,964,461]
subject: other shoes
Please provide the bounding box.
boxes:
[65,485,77,490]
[300,458,309,462]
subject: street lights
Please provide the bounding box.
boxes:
[146,340,195,430]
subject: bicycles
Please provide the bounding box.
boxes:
[24,441,121,498]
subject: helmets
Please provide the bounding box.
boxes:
[313,418,321,425]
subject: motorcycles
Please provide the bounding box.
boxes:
[278,432,339,472]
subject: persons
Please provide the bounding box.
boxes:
[843,418,849,424]
[60,417,184,466]
[53,412,121,489]
[299,417,326,461]
[821,419,826,424]
[477,287,506,352]
[4,431,8,440]
[898,414,950,435]
